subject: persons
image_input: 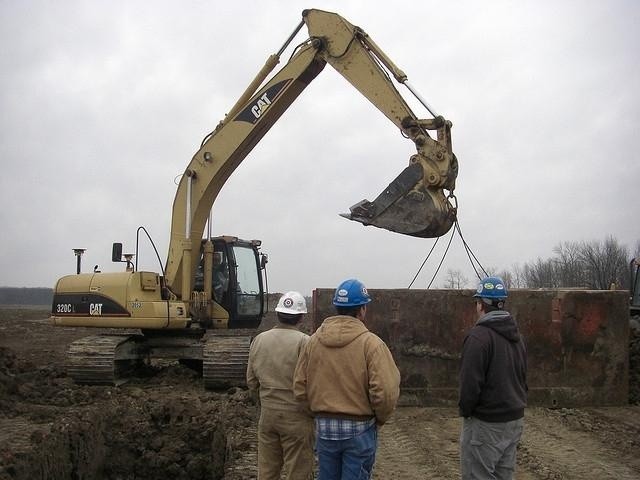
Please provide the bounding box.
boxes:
[458,277,528,480]
[293,280,401,480]
[246,291,317,480]
[216,262,228,288]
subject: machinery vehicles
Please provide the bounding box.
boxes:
[50,6,458,394]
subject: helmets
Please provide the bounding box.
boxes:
[275,291,308,315]
[472,277,508,298]
[333,279,372,307]
